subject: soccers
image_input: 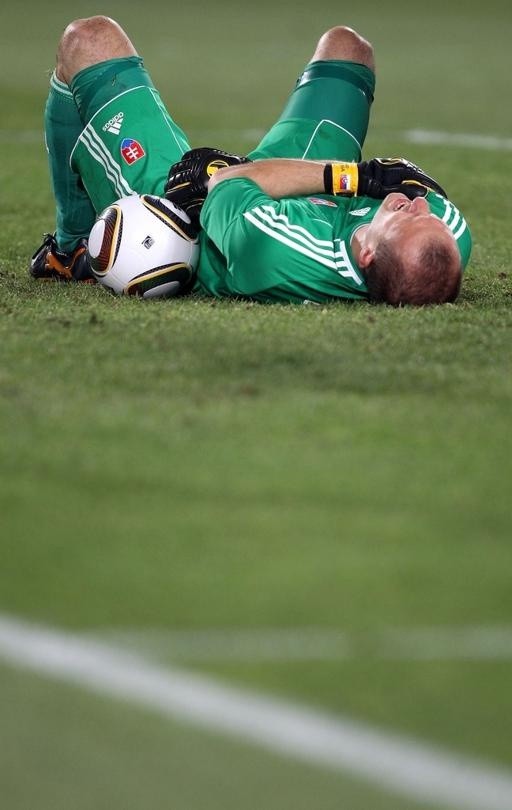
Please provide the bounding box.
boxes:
[88,194,200,300]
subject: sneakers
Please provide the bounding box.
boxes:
[29,231,98,284]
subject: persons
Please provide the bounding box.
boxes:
[27,14,473,310]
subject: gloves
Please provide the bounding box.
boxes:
[326,157,448,201]
[165,147,254,215]
